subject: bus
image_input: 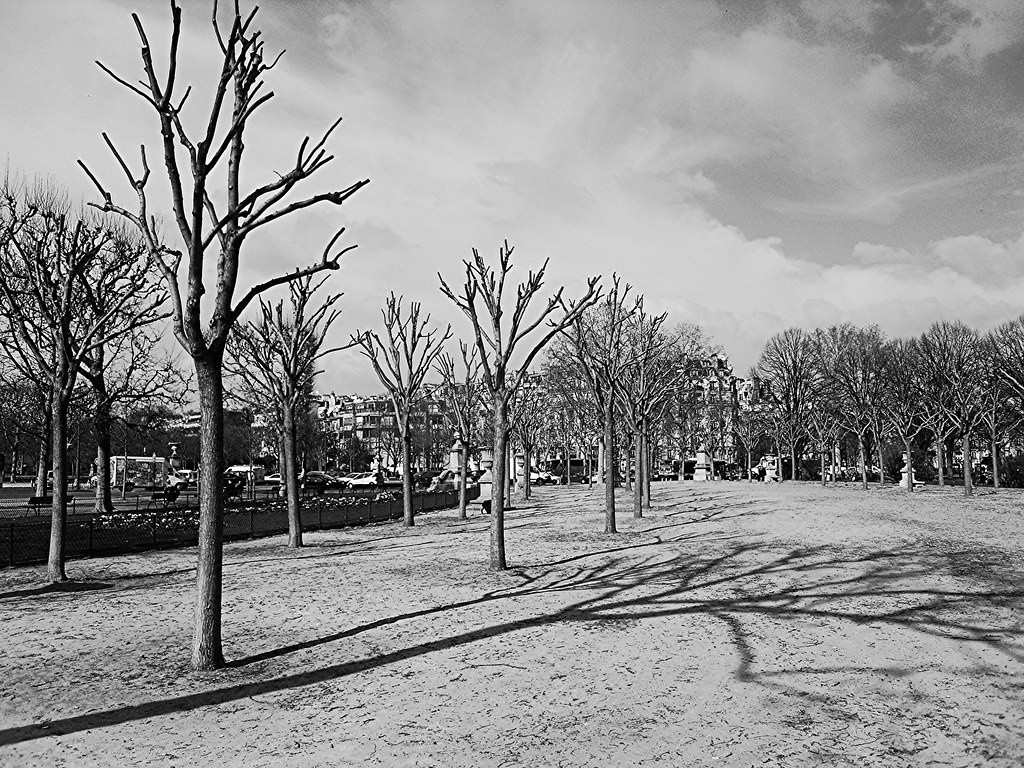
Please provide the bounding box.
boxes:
[541,459,584,474]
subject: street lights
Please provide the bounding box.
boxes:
[377,407,387,489]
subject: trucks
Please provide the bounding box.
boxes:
[224,466,264,483]
[110,456,189,492]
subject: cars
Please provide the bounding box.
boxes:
[73,476,93,489]
[223,473,244,495]
[345,472,382,490]
[400,471,440,489]
[530,466,549,486]
[586,473,599,482]
[551,471,588,484]
[548,472,562,485]
[336,473,360,487]
[844,466,880,474]
[297,474,337,489]
[264,473,281,484]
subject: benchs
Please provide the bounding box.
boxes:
[302,479,349,495]
[223,486,243,502]
[25,494,74,516]
[148,491,180,510]
